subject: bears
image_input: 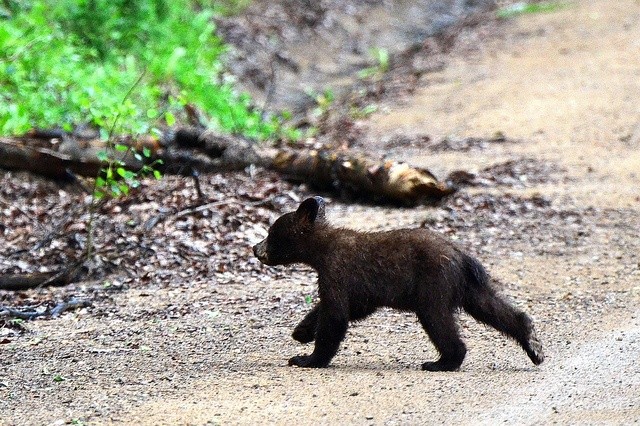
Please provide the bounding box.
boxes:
[251,194,548,372]
